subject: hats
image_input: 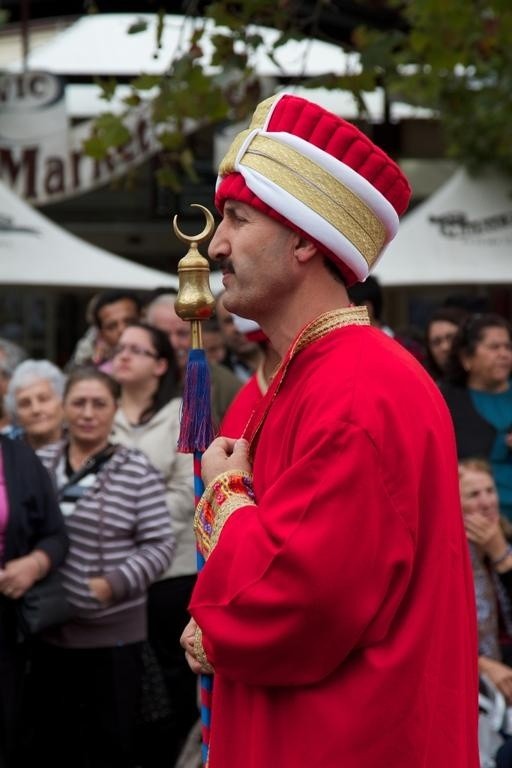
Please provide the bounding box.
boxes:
[215,92,414,286]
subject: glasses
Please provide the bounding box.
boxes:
[118,344,158,359]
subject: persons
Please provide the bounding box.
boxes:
[179,89,481,768]
